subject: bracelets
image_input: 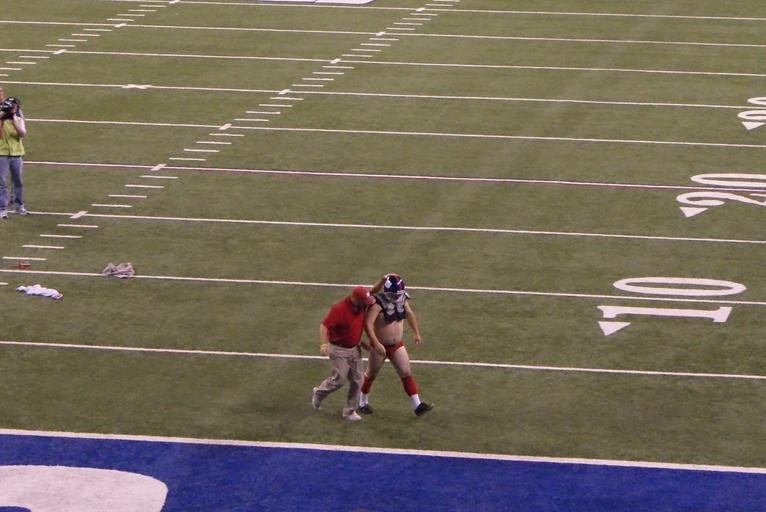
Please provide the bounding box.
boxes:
[319,343,327,347]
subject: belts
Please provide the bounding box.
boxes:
[329,340,357,350]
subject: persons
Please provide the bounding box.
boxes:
[0,96,30,219]
[311,272,399,422]
[0,87,24,118]
[358,273,436,417]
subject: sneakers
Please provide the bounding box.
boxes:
[2,206,30,222]
[341,404,373,421]
[414,401,435,417]
[311,386,323,410]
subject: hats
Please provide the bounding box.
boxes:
[4,95,20,107]
[353,285,377,306]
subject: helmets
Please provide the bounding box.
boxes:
[384,276,405,301]
